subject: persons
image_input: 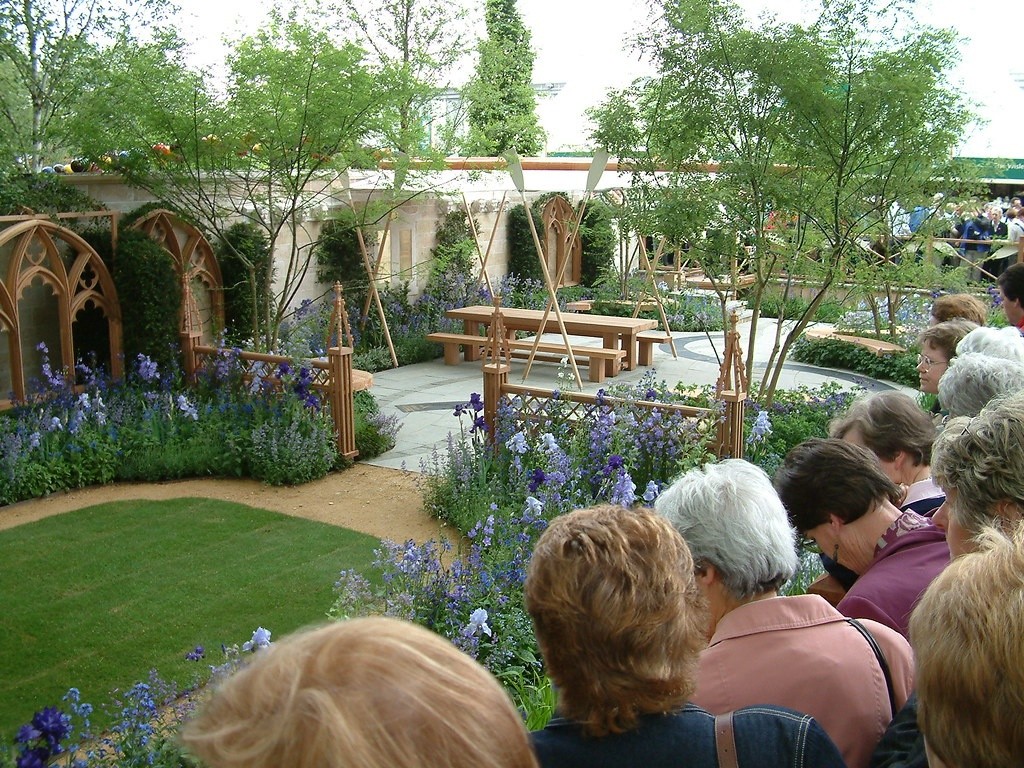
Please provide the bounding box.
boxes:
[180,191,1024,768]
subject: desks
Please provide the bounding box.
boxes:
[445,306,658,377]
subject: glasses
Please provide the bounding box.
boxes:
[960,417,988,455]
[799,531,821,553]
[917,354,946,371]
[941,415,952,427]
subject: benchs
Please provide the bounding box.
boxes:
[506,326,672,365]
[565,292,680,310]
[427,333,626,382]
[806,326,909,355]
[312,368,373,394]
